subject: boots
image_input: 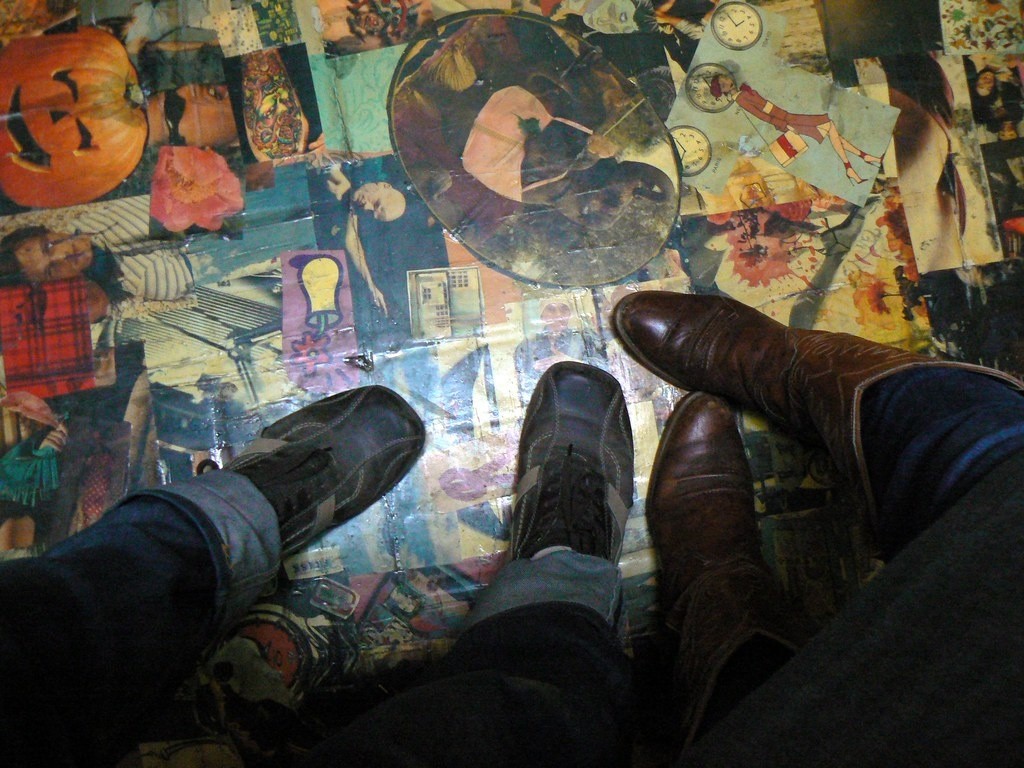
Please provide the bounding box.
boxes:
[611,293,1024,541]
[645,389,814,768]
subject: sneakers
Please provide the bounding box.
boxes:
[506,360,634,567]
[207,386,424,557]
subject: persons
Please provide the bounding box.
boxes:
[1,286,1021,767]
[1,198,201,302]
[301,147,409,318]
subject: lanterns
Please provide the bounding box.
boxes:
[1,27,150,208]
